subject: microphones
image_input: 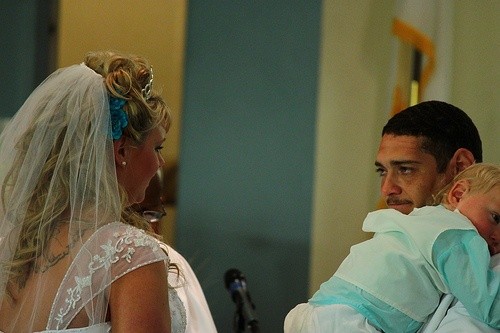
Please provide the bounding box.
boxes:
[224,269,258,326]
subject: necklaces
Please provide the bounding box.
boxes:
[57,218,102,225]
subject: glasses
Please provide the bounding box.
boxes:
[137,204,167,223]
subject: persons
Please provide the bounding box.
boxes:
[129,175,217,333]
[285,163,500,333]
[376,100,500,333]
[0,50,171,333]
